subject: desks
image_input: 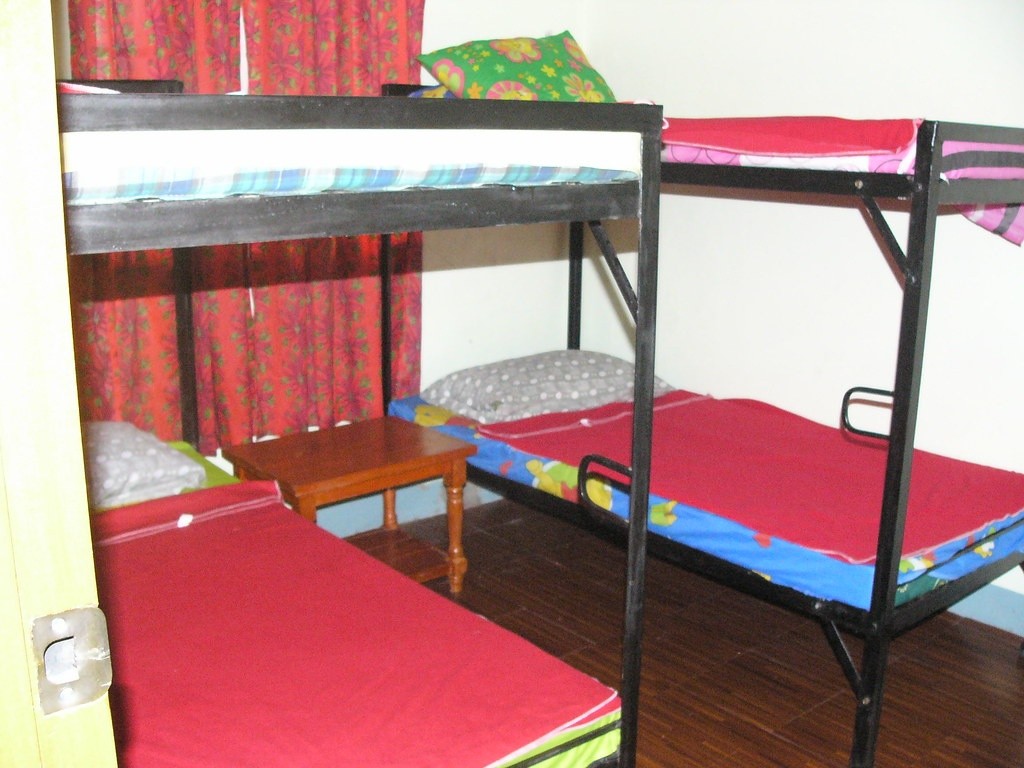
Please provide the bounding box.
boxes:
[223,415,478,593]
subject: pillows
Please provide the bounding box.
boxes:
[416,30,617,103]
[82,421,206,511]
[419,349,676,425]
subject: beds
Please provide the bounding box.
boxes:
[382,85,1024,768]
[56,78,664,768]
